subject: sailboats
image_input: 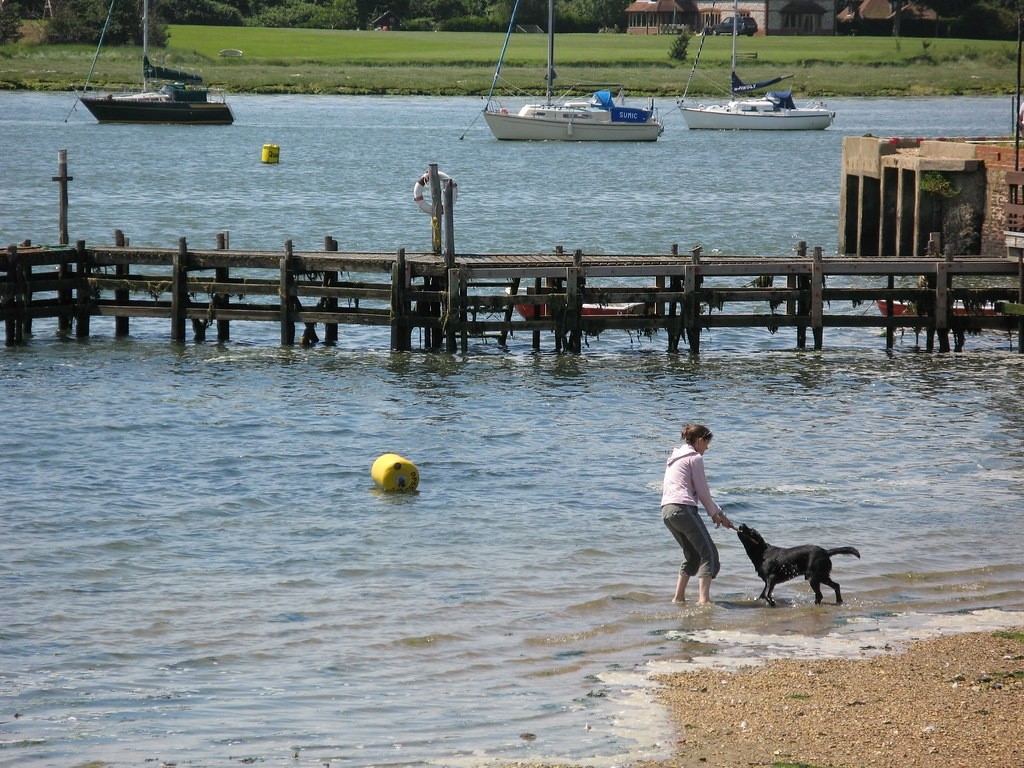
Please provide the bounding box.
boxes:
[481,0,664,142]
[680,0,835,131]
[77,0,235,126]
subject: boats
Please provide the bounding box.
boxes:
[505,287,648,315]
[874,297,999,321]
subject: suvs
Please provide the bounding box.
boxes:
[712,15,758,36]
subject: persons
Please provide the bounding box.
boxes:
[661,424,734,605]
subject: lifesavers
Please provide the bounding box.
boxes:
[413,172,460,215]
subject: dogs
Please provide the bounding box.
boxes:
[738,522,861,607]
